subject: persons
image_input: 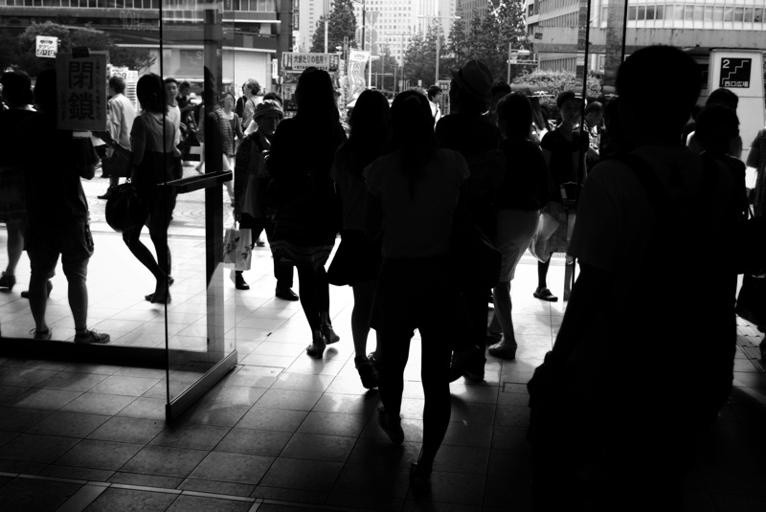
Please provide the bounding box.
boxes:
[96,76,138,201]
[9,71,113,347]
[165,46,765,511]
[428,126,437,130]
[119,72,178,306]
[1,67,57,300]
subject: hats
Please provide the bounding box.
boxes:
[253,99,284,120]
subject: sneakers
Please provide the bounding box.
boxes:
[145,274,172,305]
[354,350,487,478]
[32,328,108,343]
[0,269,51,298]
[533,288,557,301]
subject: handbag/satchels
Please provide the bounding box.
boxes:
[105,179,149,231]
[223,219,252,270]
[528,213,560,263]
[736,274,766,328]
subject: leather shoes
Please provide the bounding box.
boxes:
[276,287,298,300]
[98,194,108,200]
[230,273,249,289]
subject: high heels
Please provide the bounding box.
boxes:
[487,327,517,360]
[307,322,339,359]
